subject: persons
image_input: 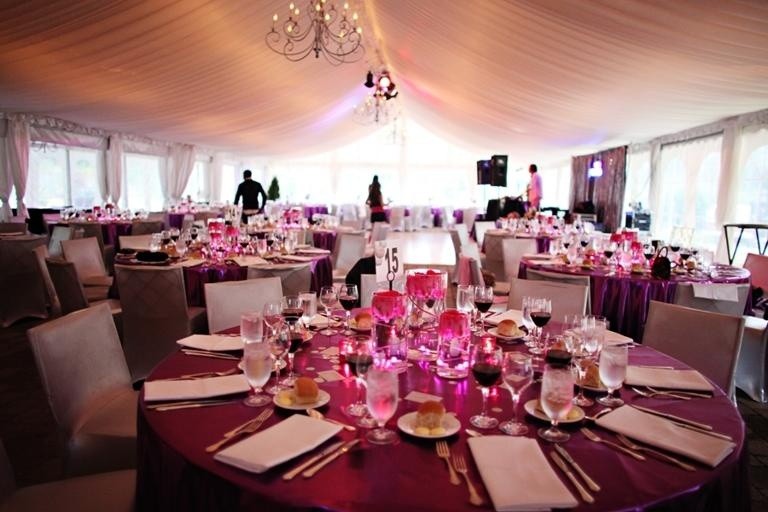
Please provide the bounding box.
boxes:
[526,164,543,211]
[365,184,392,222]
[234,170,266,224]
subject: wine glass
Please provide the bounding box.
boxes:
[502,207,566,236]
[469,342,504,430]
[343,337,373,379]
[537,364,577,443]
[265,322,293,396]
[60,208,144,224]
[239,282,629,446]
[364,367,402,446]
[241,347,270,407]
[500,351,532,435]
[593,349,628,409]
[149,218,297,267]
[549,235,713,273]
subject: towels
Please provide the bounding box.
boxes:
[468,437,580,512]
[214,415,345,476]
[595,403,736,469]
[143,374,251,400]
[623,364,715,391]
[174,333,243,352]
[602,328,632,347]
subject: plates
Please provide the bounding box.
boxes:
[399,411,465,439]
[272,387,331,411]
[522,397,586,423]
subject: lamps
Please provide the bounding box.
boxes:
[264,0,365,67]
[365,34,398,122]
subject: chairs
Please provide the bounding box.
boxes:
[204,276,284,334]
[27,302,143,475]
[641,299,747,398]
[2,440,138,512]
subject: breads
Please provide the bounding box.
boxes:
[355,311,372,328]
[576,360,601,388]
[414,399,447,428]
[496,319,518,337]
[293,377,320,404]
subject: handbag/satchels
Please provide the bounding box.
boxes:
[652,246,671,279]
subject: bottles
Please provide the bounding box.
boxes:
[104,193,114,215]
[92,199,101,217]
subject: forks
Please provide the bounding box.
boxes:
[450,447,483,506]
[434,441,461,488]
[159,361,239,381]
[579,427,647,465]
[207,404,274,455]
[616,432,697,474]
[632,383,713,405]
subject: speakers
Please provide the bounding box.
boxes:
[490,154,508,186]
[477,159,491,184]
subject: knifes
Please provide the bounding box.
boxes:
[637,405,733,445]
[283,439,345,480]
[558,444,605,494]
[303,439,357,478]
[147,400,227,411]
[545,444,596,504]
